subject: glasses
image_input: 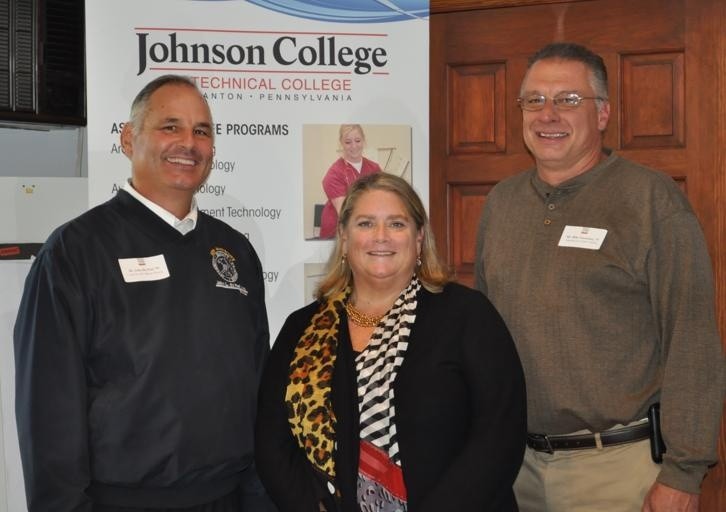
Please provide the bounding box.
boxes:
[517,93,605,112]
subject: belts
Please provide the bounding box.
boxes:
[525,421,651,454]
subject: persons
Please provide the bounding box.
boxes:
[255,170,529,511]
[473,43,724,512]
[316,124,384,242]
[10,76,269,512]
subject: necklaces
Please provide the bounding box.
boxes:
[345,296,384,330]
